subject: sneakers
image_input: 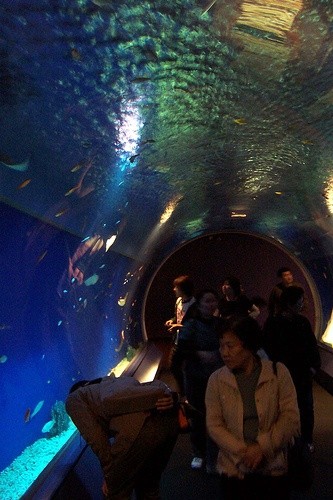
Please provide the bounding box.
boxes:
[206,465,217,474]
[191,457,203,468]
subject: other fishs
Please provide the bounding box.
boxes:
[0,0,333,433]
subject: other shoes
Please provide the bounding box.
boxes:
[305,443,315,457]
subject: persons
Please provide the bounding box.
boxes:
[65,267,322,500]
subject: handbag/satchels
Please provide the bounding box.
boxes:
[99,376,171,416]
